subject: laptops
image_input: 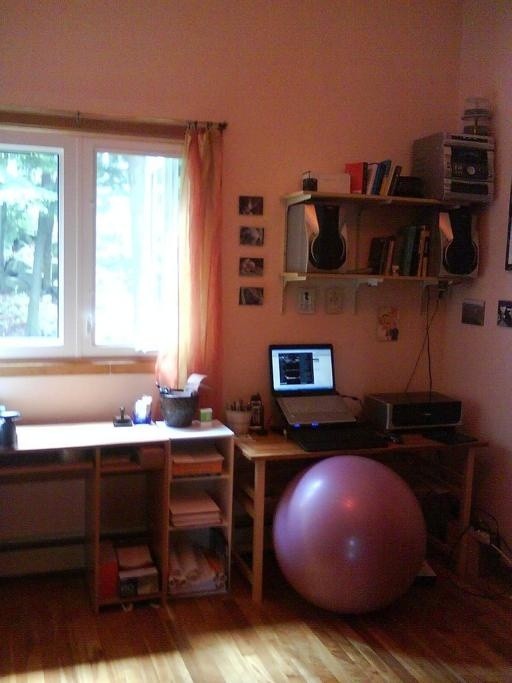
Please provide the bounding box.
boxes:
[269,343,358,426]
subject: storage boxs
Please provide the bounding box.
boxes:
[302,174,351,193]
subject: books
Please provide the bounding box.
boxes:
[172,446,224,477]
[169,488,223,529]
[116,544,159,598]
[100,443,164,466]
[368,222,431,278]
[343,158,403,196]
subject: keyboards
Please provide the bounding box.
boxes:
[294,428,388,451]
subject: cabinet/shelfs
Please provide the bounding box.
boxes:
[0,419,236,615]
[282,191,470,281]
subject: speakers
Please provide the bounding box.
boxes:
[287,201,358,274]
[419,211,479,277]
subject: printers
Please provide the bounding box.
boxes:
[364,389,467,434]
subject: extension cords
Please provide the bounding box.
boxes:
[469,523,493,547]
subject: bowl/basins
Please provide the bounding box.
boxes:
[159,389,199,427]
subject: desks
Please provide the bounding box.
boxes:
[232,431,488,603]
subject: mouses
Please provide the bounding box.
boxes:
[386,433,404,445]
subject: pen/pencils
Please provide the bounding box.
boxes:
[156,379,173,395]
[227,398,251,412]
